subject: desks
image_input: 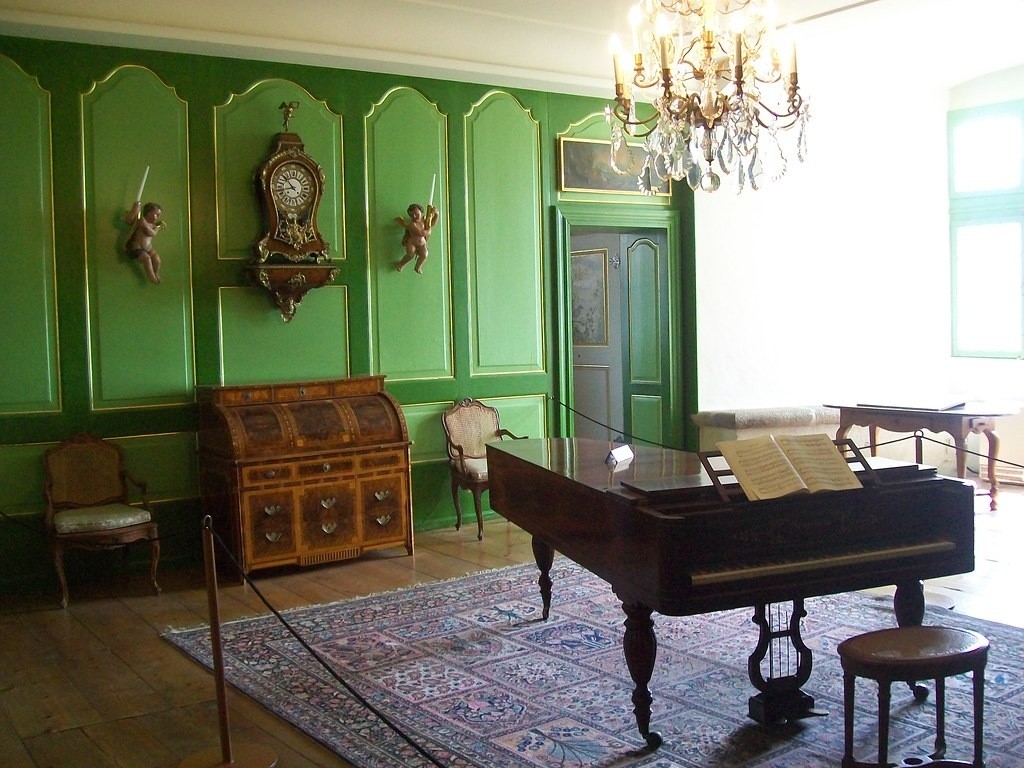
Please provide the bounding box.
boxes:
[823,401,1016,512]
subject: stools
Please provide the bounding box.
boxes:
[835,624,991,768]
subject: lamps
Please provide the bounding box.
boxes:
[603,0,812,200]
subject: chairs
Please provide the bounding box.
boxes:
[442,397,530,541]
[43,430,163,610]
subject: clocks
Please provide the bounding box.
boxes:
[240,131,338,324]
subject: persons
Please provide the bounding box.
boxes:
[397,204,440,274]
[126,202,163,284]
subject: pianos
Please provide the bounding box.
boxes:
[484,435,977,750]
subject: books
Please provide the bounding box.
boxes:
[716,432,864,502]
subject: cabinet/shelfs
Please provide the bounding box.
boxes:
[194,373,415,584]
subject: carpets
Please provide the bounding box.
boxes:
[151,554,1024,768]
